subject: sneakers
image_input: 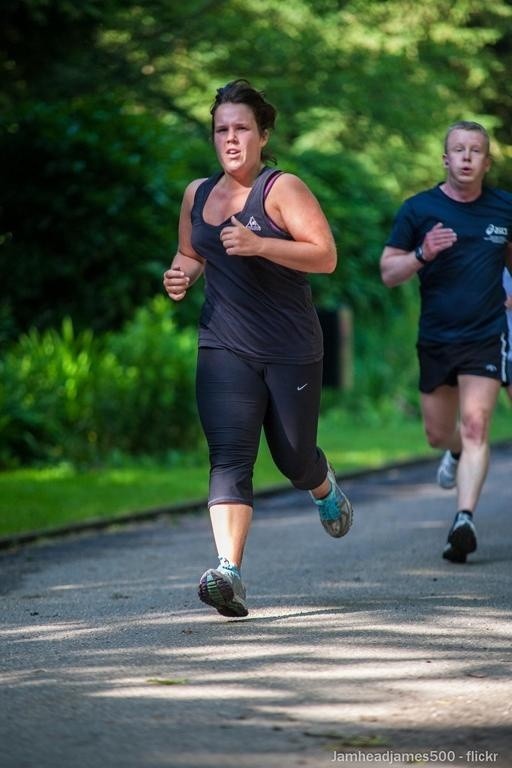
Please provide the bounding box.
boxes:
[198,557,249,618]
[308,462,354,538]
[441,513,478,564]
[437,449,459,490]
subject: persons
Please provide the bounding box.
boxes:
[380,121,512,563]
[163,80,353,617]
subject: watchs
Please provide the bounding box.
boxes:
[416,246,427,264]
